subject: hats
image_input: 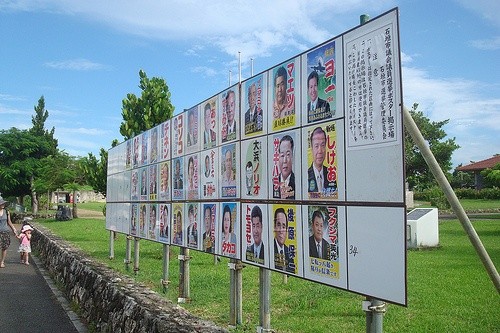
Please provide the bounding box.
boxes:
[0,196,9,205]
[22,225,34,232]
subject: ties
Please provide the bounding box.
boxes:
[311,105,315,122]
[255,249,257,263]
[250,114,253,121]
[318,174,322,191]
[317,243,321,256]
[280,250,283,265]
[229,127,231,133]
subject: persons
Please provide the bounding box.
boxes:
[307,127,337,194]
[273,208,296,274]
[126,103,216,254]
[246,162,253,196]
[309,210,331,260]
[245,84,263,134]
[272,66,296,120]
[246,205,264,265]
[222,150,236,187]
[16,225,34,265]
[221,205,237,256]
[272,134,295,200]
[222,90,236,143]
[0,196,17,267]
[307,71,332,123]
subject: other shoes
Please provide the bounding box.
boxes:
[25,262,29,264]
[0,264,5,267]
[21,260,24,263]
